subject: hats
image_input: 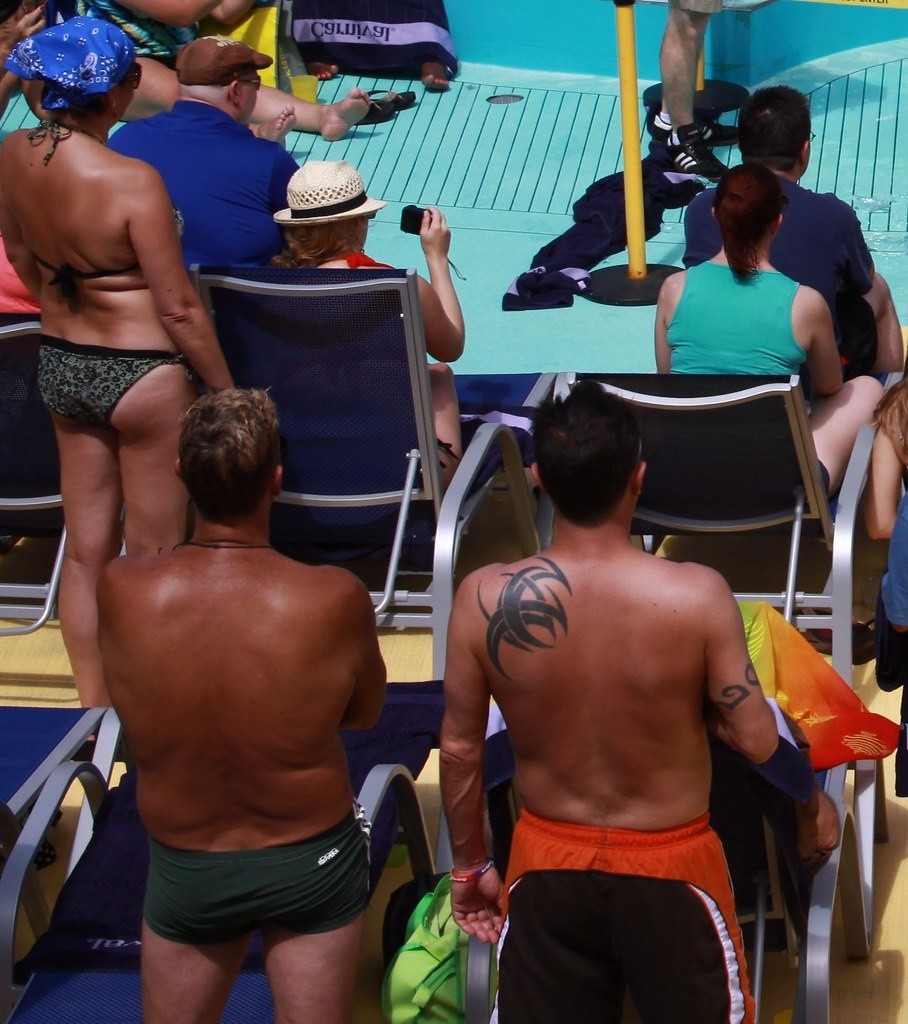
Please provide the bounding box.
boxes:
[273,159,387,225]
[176,36,274,85]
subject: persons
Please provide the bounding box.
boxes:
[273,159,466,486]
[650,0,738,182]
[862,375,908,797]
[654,161,885,498]
[95,388,388,1024]
[438,389,840,1024]
[0,16,236,740]
[0,0,457,152]
[682,84,905,374]
[106,35,299,271]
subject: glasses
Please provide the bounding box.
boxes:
[779,195,788,211]
[368,213,376,219]
[222,76,261,91]
[119,63,142,89]
[808,131,816,142]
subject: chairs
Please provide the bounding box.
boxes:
[0,263,885,1024]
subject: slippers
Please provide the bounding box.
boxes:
[352,100,396,124]
[366,90,415,110]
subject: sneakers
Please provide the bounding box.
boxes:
[666,123,731,183]
[653,111,740,146]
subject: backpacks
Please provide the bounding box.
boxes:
[378,873,498,1024]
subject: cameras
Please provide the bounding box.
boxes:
[400,205,431,235]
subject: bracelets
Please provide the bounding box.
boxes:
[450,858,494,882]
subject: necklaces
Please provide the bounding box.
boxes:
[173,542,273,551]
